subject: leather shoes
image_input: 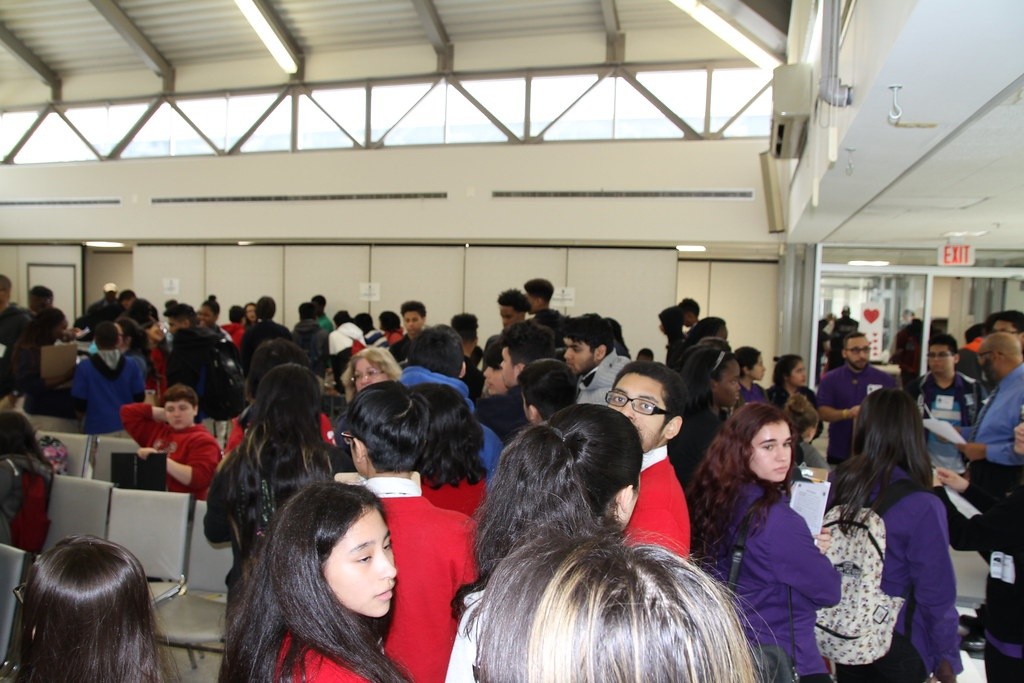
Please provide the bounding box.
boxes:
[959,631,986,652]
[959,614,981,631]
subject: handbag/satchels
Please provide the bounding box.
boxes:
[738,645,798,683]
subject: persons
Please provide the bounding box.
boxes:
[1,273,1024,683]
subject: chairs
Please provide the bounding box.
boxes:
[0,428,234,683]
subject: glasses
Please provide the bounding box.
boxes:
[12,582,26,605]
[604,390,675,418]
[926,350,951,358]
[844,346,873,354]
[349,368,383,385]
[977,350,1003,366]
[341,430,355,445]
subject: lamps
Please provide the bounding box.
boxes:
[233,0,301,75]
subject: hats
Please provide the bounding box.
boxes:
[102,282,118,293]
[126,299,153,319]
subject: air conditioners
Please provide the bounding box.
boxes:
[768,61,813,159]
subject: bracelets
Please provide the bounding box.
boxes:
[841,409,849,420]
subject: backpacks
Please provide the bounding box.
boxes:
[809,481,935,666]
[196,337,244,422]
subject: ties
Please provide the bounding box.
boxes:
[966,384,1000,442]
